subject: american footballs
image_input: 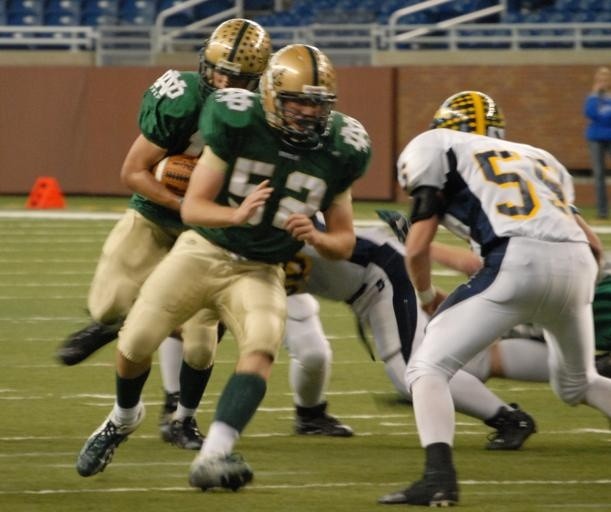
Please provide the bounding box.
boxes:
[151,155,199,195]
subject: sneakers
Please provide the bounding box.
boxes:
[57,323,119,365]
[293,414,354,436]
[377,468,460,507]
[75,401,145,477]
[186,453,253,490]
[484,402,536,450]
[159,384,205,451]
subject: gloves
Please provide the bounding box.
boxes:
[375,209,409,244]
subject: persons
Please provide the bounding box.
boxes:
[158,290,354,443]
[281,210,611,451]
[380,90,611,508]
[582,66,610,218]
[58,18,271,450]
[375,210,611,348]
[76,44,370,492]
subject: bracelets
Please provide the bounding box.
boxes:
[416,285,436,304]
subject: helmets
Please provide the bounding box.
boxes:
[259,43,338,151]
[428,90,505,140]
[197,18,271,100]
